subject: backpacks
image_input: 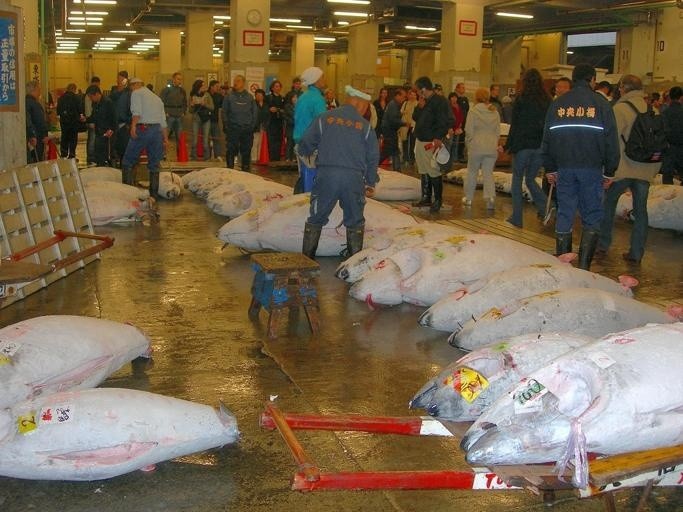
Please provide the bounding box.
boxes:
[618,100,666,163]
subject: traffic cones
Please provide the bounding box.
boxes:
[378,134,389,165]
[255,131,272,167]
[197,128,203,160]
[281,128,286,161]
[46,140,57,159]
[140,148,149,166]
[176,132,190,161]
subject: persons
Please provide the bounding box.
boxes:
[293,67,329,193]
[491,68,682,229]
[597,74,663,265]
[541,65,620,271]
[299,85,380,260]
[374,76,470,213]
[26,72,299,200]
[323,89,339,112]
[462,88,500,210]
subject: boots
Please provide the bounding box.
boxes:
[555,230,573,257]
[122,166,137,186]
[148,172,160,202]
[581,230,600,269]
[340,226,366,261]
[302,222,321,257]
[411,173,433,207]
[429,175,443,213]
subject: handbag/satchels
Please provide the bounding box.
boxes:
[198,108,210,122]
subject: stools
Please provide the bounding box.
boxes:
[248,251,321,340]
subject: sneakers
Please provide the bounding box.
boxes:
[621,252,641,265]
[543,207,557,228]
[487,197,495,211]
[506,216,523,229]
[461,196,473,207]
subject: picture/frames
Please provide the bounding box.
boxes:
[459,20,477,36]
[243,31,264,46]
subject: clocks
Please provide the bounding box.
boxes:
[246,8,262,27]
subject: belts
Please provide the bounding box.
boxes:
[168,113,181,118]
[136,123,161,126]
